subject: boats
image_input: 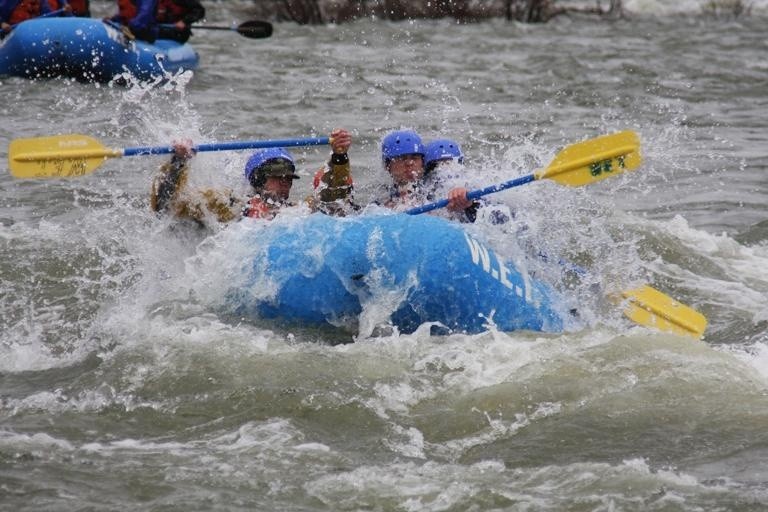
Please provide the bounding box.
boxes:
[1,17,200,83]
[196,213,583,337]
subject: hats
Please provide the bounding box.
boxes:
[248,156,301,187]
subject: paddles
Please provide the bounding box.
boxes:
[392,129,641,215]
[184,19,273,39]
[481,208,709,345]
[8,130,343,180]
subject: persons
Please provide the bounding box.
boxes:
[111,0,205,44]
[0,0,91,40]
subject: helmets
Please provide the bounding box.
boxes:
[381,129,425,168]
[314,164,354,188]
[423,138,464,171]
[243,144,295,180]
